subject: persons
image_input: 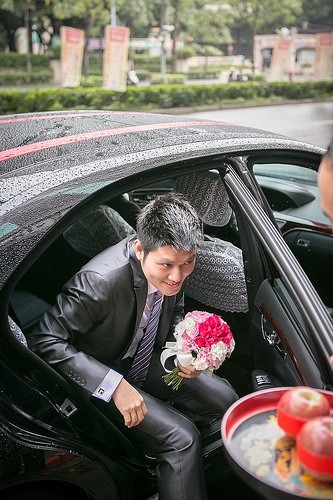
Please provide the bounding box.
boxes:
[27,192,242,499]
[316,139,333,223]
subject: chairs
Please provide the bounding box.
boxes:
[168,171,248,341]
[60,200,134,258]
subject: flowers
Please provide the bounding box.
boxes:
[155,309,236,394]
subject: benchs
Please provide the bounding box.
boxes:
[4,292,51,346]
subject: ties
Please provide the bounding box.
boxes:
[126,290,162,388]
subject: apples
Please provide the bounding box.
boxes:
[278,386,329,438]
[296,416,333,483]
